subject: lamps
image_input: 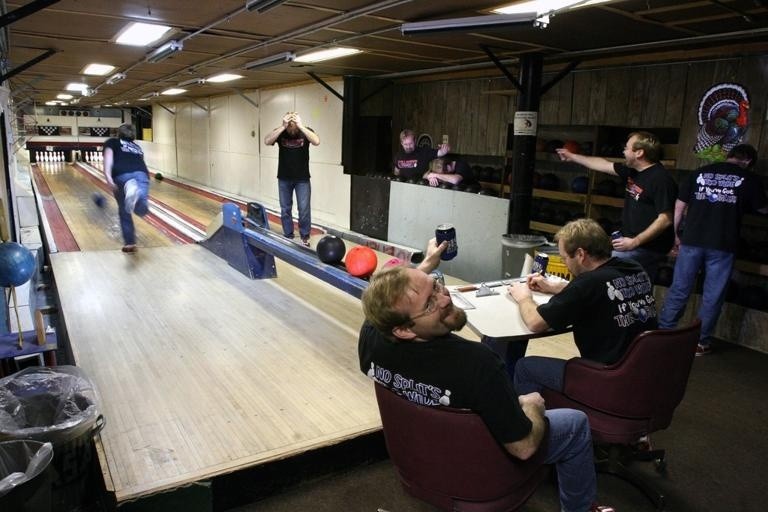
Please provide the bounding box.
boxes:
[146,41,183,65]
[179,77,206,90]
[400,11,550,36]
[140,91,161,99]
[106,73,126,85]
[245,51,296,71]
[248,2,275,12]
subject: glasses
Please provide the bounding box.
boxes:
[410,274,445,320]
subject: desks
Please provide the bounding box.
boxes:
[444,274,583,369]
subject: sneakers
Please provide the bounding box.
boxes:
[695,343,712,357]
[300,237,310,247]
[121,246,137,254]
[123,179,139,214]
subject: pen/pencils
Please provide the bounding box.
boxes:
[529,269,543,284]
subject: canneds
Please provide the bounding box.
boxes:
[611,230,623,240]
[532,251,549,277]
[435,224,458,261]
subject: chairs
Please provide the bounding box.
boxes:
[373,378,553,511]
[527,317,703,510]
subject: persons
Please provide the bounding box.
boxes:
[263,110,321,248]
[102,123,151,254]
[393,129,448,178]
[554,130,678,279]
[657,144,768,357]
[422,155,481,187]
[506,217,659,397]
[357,237,616,512]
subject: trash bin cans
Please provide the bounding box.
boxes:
[502,233,547,281]
[0,392,106,512]
[534,246,574,283]
